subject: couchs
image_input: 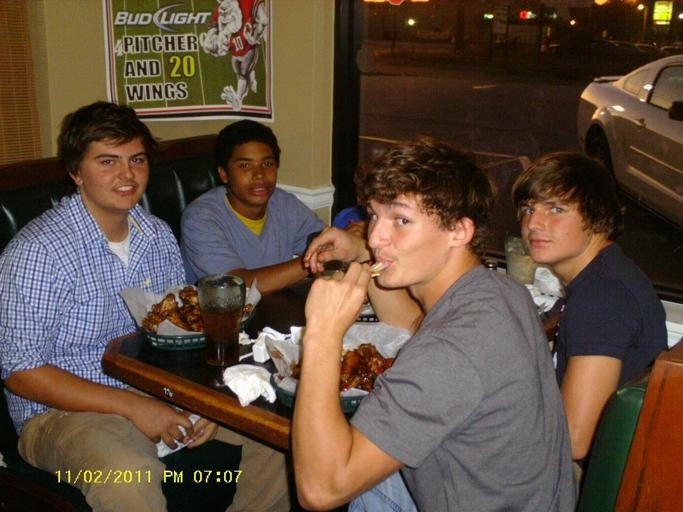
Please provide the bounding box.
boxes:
[0,133,242,512]
[574,337,682,511]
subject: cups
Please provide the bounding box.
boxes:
[504,234,538,285]
[197,273,246,366]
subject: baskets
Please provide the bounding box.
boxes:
[357,313,379,322]
[145,311,257,352]
[270,374,368,413]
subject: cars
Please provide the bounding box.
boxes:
[609,41,683,72]
[577,54,683,233]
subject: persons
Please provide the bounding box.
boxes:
[288,132,578,512]
[509,149,670,475]
[0,101,300,512]
[175,119,371,305]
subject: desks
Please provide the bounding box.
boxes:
[100,263,567,452]
